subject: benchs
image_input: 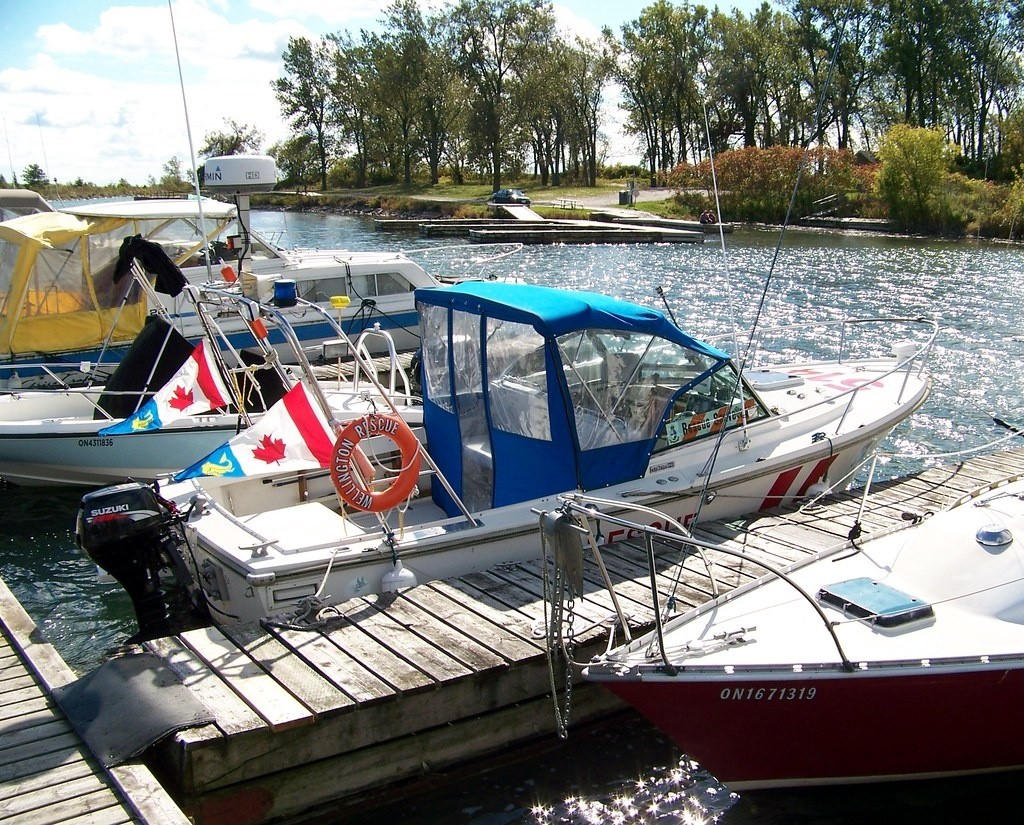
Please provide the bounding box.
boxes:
[552,202,583,210]
[460,433,493,470]
[489,375,630,451]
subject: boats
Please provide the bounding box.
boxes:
[526,479,1024,793]
[0,158,529,390]
[0,281,603,492]
[0,189,211,291]
[74,281,937,631]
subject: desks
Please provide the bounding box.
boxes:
[558,200,581,210]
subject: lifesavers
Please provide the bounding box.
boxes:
[330,413,422,512]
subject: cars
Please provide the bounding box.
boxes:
[491,188,531,205]
